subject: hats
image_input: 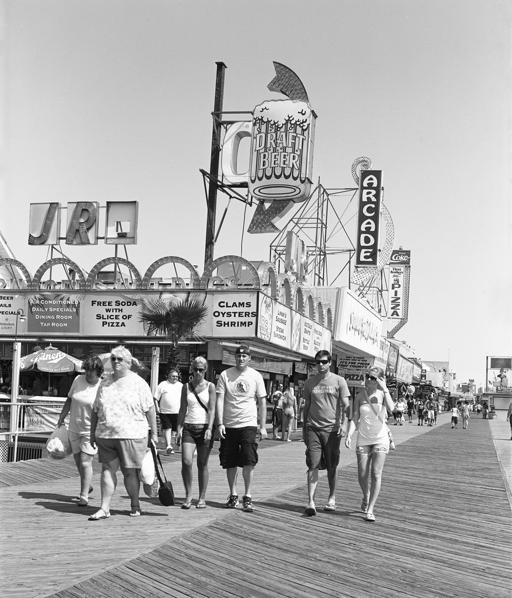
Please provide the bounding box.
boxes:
[236,345,250,354]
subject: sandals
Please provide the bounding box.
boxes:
[78,498,88,506]
[78,486,94,507]
[130,506,140,516]
[89,509,110,519]
[182,500,192,509]
[196,502,206,508]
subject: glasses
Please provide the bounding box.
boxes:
[112,358,123,361]
[365,374,375,379]
[316,361,330,364]
[193,369,205,372]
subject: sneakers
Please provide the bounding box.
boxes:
[305,506,316,515]
[324,505,336,511]
[167,446,172,454]
[227,498,252,510]
[360,498,375,521]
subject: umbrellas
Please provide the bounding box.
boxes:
[21,343,85,391]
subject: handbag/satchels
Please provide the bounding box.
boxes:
[159,481,174,507]
[388,431,395,450]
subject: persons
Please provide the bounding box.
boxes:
[0,371,44,396]
[271,383,305,441]
[303,350,396,522]
[57,346,267,520]
[494,368,507,393]
[397,393,512,442]
